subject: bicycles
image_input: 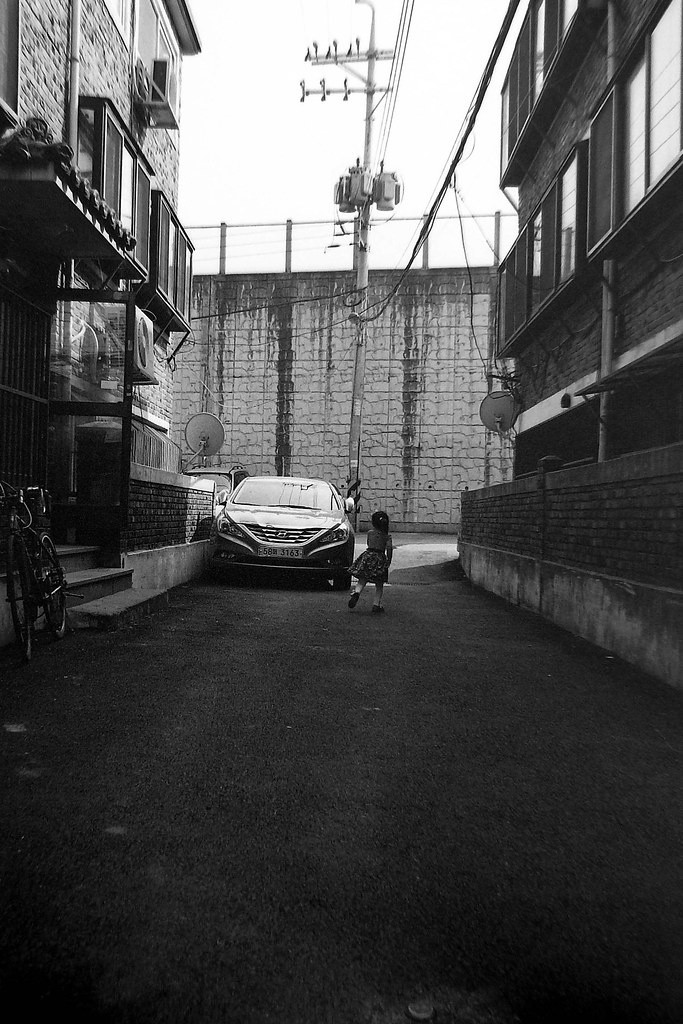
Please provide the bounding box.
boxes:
[0,479,87,663]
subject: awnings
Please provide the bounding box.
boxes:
[130,282,192,363]
[73,247,147,297]
[574,331,683,448]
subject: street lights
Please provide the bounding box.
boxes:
[347,313,367,529]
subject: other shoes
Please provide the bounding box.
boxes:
[372,605,385,612]
[348,593,359,608]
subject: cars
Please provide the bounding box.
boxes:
[207,474,356,593]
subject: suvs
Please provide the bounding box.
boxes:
[182,463,251,505]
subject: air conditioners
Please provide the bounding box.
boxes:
[107,304,154,382]
[149,58,180,126]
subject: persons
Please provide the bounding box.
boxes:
[348,512,393,613]
